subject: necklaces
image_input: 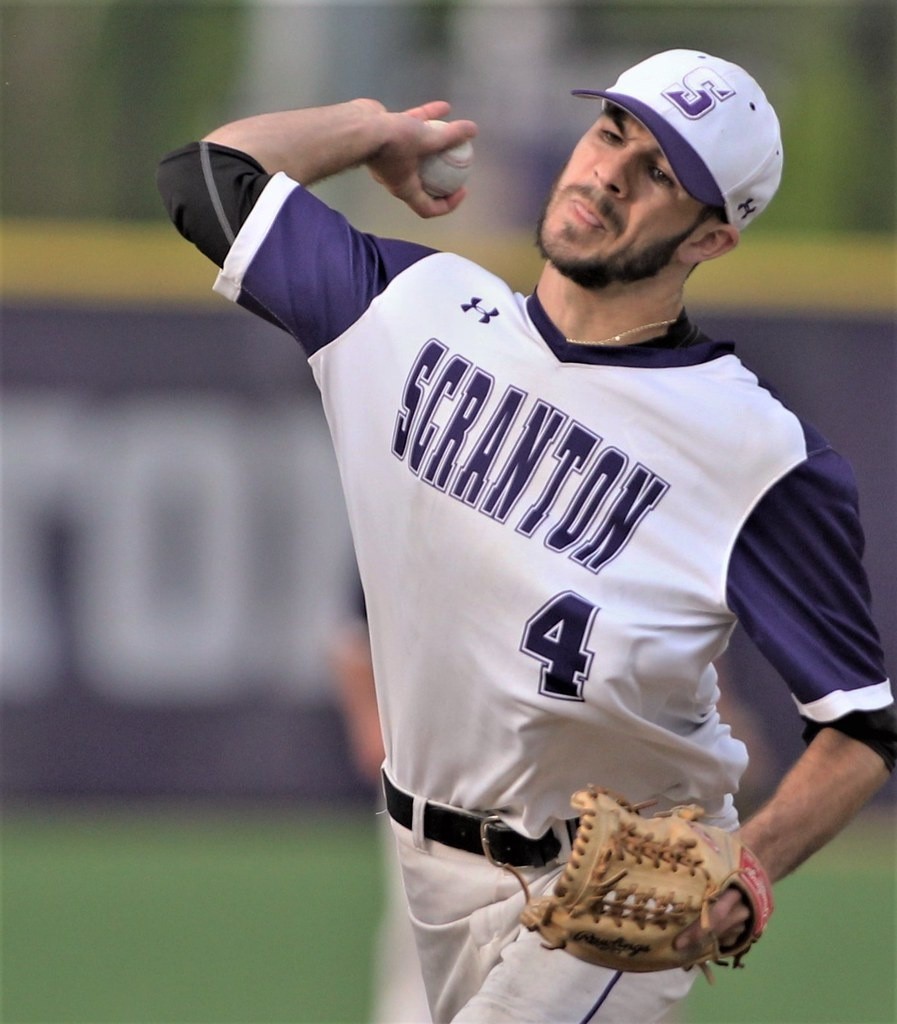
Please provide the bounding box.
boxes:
[563,318,685,349]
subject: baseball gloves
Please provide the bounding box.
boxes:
[520,781,776,988]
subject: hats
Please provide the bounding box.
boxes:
[569,49,784,231]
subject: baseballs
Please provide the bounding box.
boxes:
[417,120,475,197]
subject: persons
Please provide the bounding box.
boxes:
[331,524,778,799]
[154,46,894,1023]
